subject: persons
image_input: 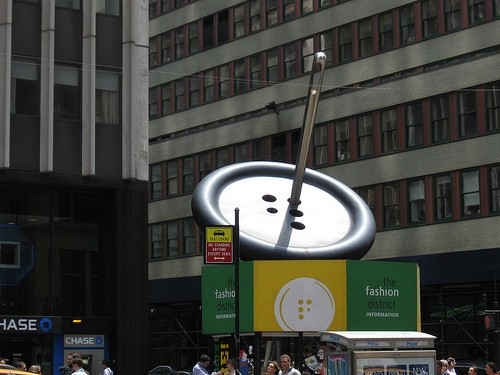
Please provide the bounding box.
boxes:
[437,357,500,375]
[192,354,319,375]
[0,352,113,375]
